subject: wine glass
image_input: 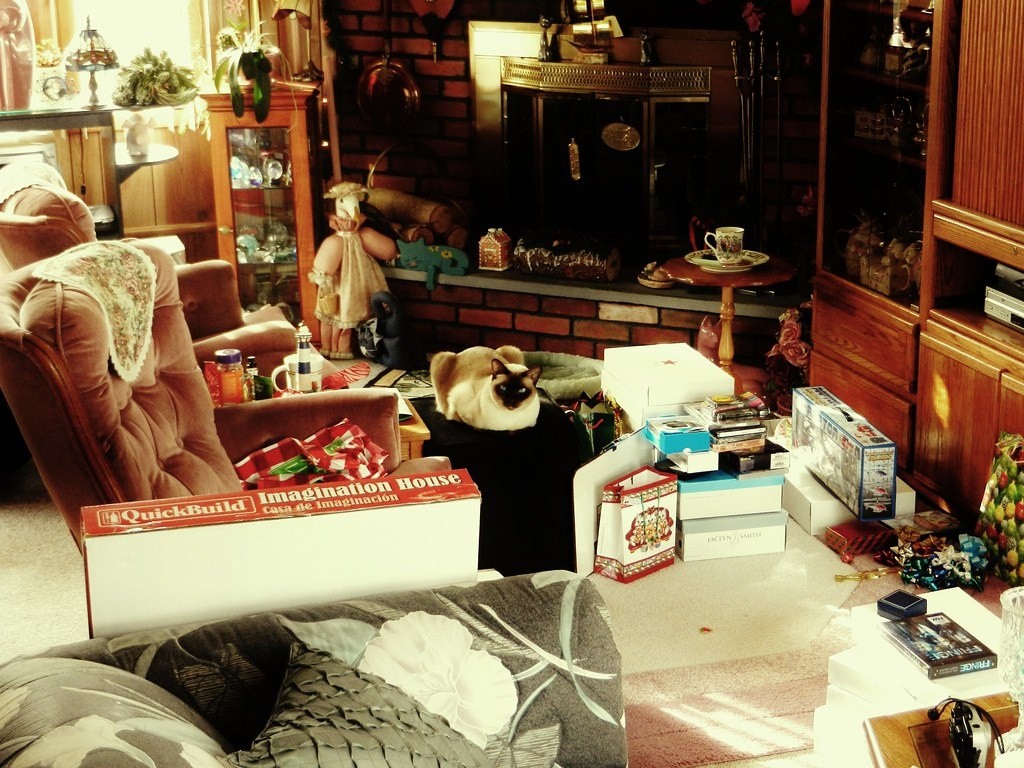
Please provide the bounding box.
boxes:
[993,586,1024,758]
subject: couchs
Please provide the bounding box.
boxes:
[0,574,634,768]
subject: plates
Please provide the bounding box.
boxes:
[601,122,640,151]
[684,249,770,272]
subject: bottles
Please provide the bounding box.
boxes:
[245,356,259,399]
[214,348,245,407]
[567,137,581,180]
[295,325,313,392]
[241,373,254,402]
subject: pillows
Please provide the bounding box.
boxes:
[215,640,494,768]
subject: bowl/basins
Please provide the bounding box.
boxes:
[994,751,1024,768]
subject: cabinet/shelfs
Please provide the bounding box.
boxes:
[801,0,958,473]
[915,0,1024,493]
[197,82,319,349]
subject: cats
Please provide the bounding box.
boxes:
[428,345,542,431]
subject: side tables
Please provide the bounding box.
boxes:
[398,397,431,460]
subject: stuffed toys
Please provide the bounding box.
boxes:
[308,181,396,359]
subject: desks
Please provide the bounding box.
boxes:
[115,140,182,186]
[0,106,124,264]
[659,258,791,376]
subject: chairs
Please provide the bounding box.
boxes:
[0,232,482,639]
[1,155,294,400]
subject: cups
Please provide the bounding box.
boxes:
[253,375,273,401]
[270,353,324,392]
[704,226,744,264]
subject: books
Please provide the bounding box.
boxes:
[682,392,768,452]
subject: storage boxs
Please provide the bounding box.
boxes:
[80,465,484,647]
[597,339,961,564]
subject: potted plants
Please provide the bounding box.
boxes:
[208,14,294,122]
[29,39,67,111]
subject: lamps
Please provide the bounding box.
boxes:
[68,18,119,109]
